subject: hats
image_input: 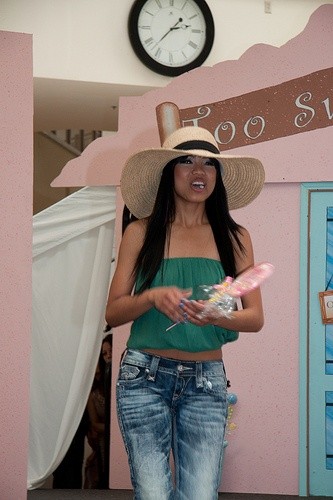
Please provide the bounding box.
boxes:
[120,126,266,219]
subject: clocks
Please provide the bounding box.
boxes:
[127,0,215,77]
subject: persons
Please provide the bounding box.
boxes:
[53,334,112,490]
[105,126,265,500]
